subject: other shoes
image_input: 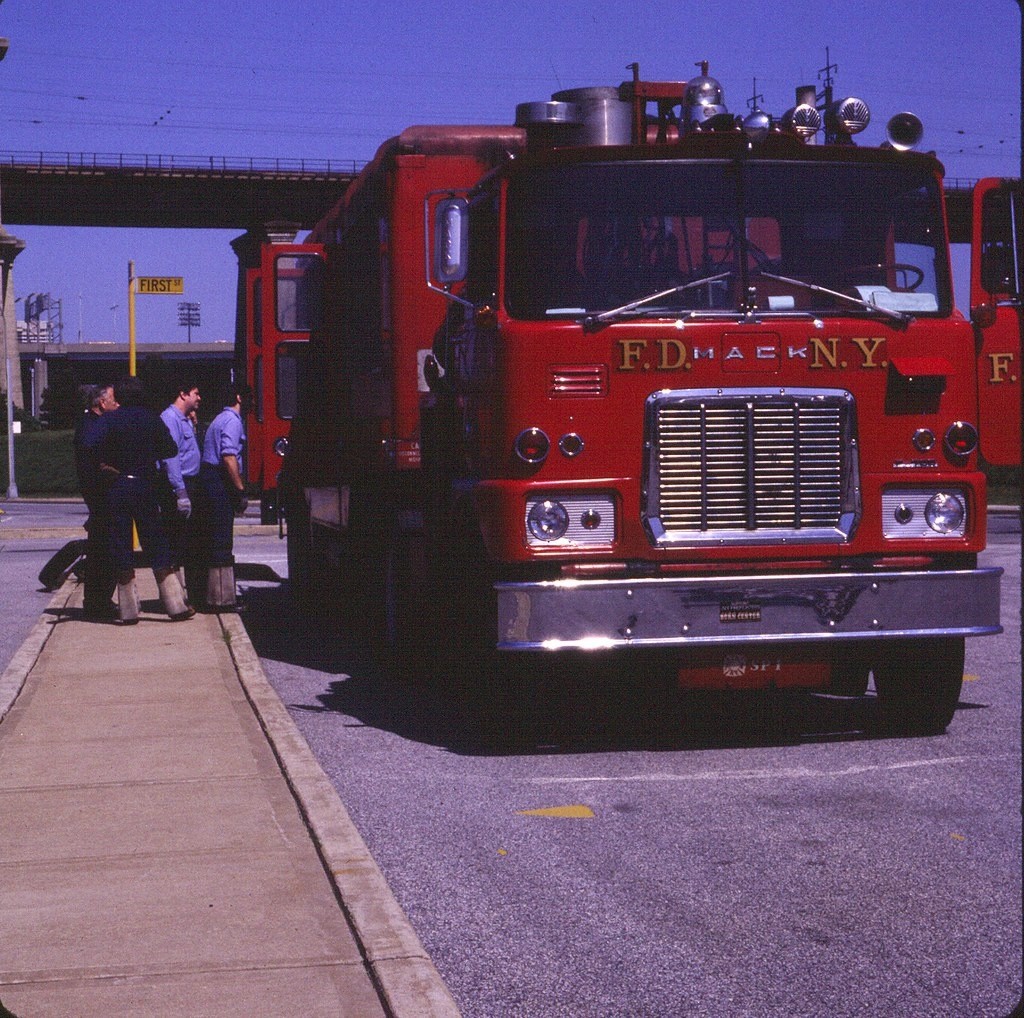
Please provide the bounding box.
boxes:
[204,604,248,613]
[123,619,137,625]
[174,608,196,621]
[84,609,119,617]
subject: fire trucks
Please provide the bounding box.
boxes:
[243,49,1022,738]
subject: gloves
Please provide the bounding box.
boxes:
[173,489,193,519]
[235,491,249,513]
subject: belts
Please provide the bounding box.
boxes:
[120,475,152,479]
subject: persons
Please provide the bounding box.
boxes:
[200,379,254,615]
[75,374,201,625]
